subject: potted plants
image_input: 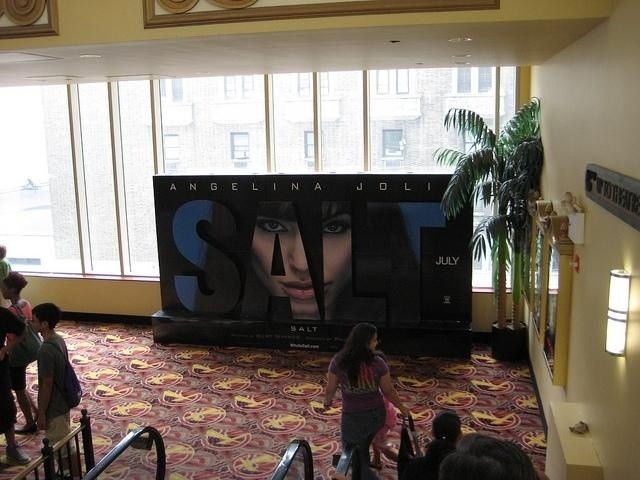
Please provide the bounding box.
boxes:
[437,96,545,364]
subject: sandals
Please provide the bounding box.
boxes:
[15,415,39,435]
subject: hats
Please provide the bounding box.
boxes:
[433,413,461,439]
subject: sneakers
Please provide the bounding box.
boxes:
[369,462,383,472]
[6,446,31,465]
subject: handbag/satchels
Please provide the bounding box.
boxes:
[8,304,42,369]
[398,414,423,480]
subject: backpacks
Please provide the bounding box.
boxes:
[43,340,82,408]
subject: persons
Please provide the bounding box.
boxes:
[29,302,85,480]
[319,320,410,480]
[367,349,401,470]
[399,410,543,480]
[247,199,355,320]
[1,242,44,473]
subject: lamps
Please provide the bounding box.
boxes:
[604,268,631,357]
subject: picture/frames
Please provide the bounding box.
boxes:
[142,0,500,29]
[0,0,59,40]
